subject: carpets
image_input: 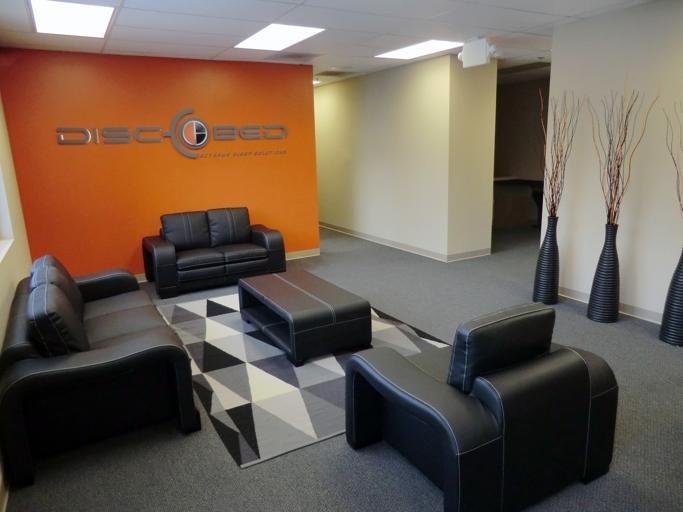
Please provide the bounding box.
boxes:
[156,285,452,469]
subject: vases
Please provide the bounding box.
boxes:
[588,223,619,323]
[660,248,683,346]
[533,217,559,304]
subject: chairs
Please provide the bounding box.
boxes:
[346,343,618,512]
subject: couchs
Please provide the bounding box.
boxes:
[142,224,286,299]
[0,268,201,491]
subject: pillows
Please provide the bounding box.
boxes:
[207,206,251,248]
[28,266,84,320]
[447,301,555,394]
[31,255,73,281]
[26,284,90,359]
[160,211,210,252]
